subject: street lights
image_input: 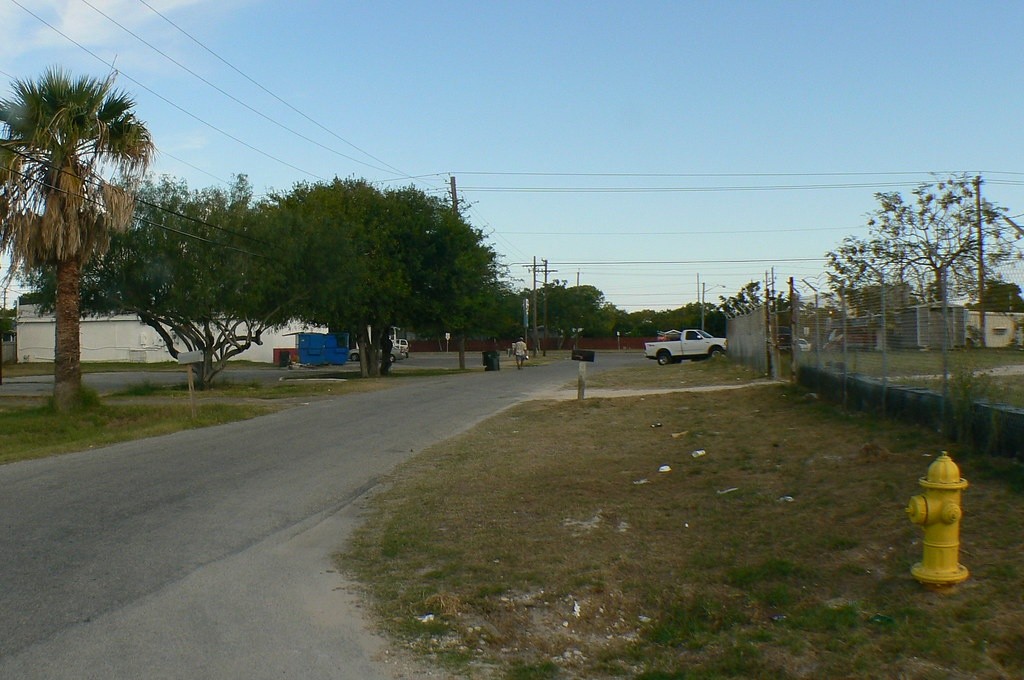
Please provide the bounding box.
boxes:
[701,284,727,332]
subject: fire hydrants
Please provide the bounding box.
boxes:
[905,450,968,585]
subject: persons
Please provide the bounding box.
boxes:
[513,338,528,369]
[381,336,393,374]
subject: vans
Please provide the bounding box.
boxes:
[393,339,409,358]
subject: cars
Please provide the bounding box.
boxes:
[347,346,404,362]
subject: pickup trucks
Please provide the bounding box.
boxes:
[644,329,729,365]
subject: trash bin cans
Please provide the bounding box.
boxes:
[483,351,500,371]
